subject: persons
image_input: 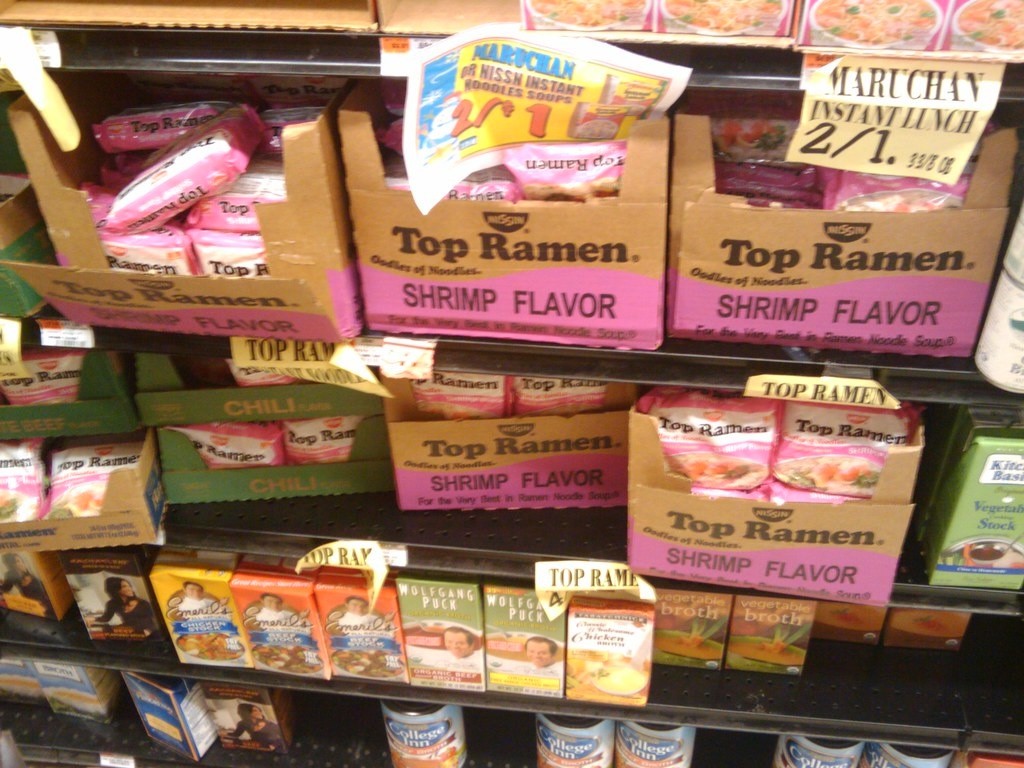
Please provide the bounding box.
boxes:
[1,552,52,618]
[173,581,225,620]
[85,576,154,641]
[250,593,305,634]
[516,635,566,697]
[427,626,484,691]
[221,702,288,753]
[339,595,384,634]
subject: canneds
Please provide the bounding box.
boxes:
[770,733,957,768]
[614,720,697,768]
[379,699,467,768]
[534,713,615,768]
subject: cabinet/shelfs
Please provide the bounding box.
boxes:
[1,34,1024,768]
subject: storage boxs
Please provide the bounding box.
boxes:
[2,1,1023,762]
